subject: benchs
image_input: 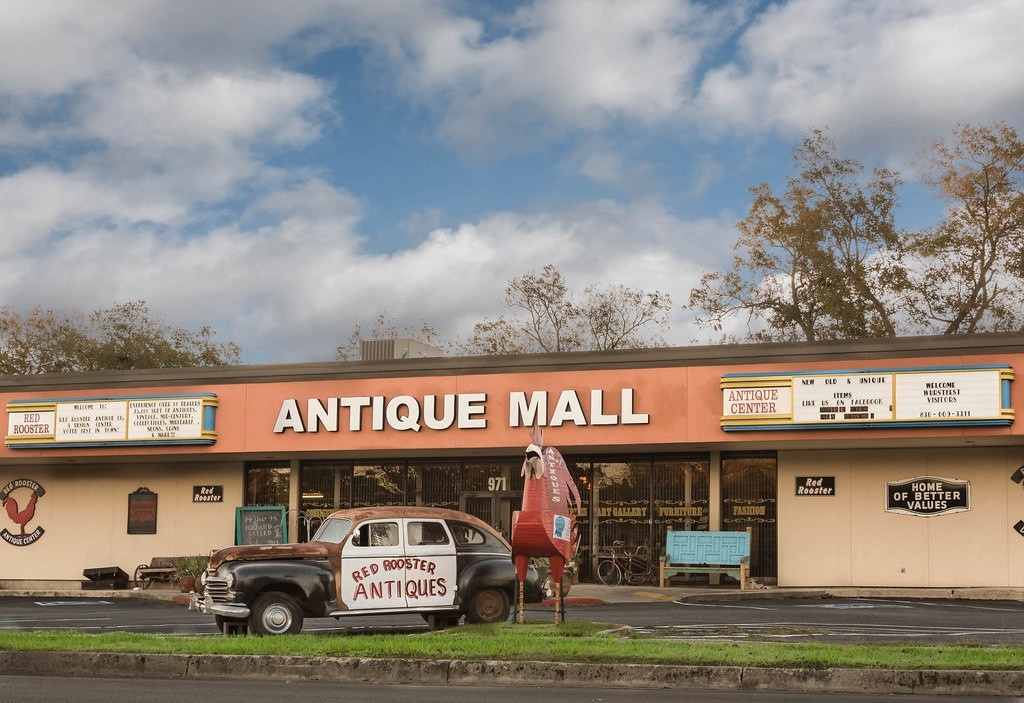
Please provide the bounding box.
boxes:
[659,526,752,591]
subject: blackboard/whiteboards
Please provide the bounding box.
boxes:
[236,506,288,545]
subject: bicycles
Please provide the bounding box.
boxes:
[597,541,666,587]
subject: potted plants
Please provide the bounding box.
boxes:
[531,554,579,597]
[169,554,209,596]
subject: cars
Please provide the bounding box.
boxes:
[188,506,543,637]
